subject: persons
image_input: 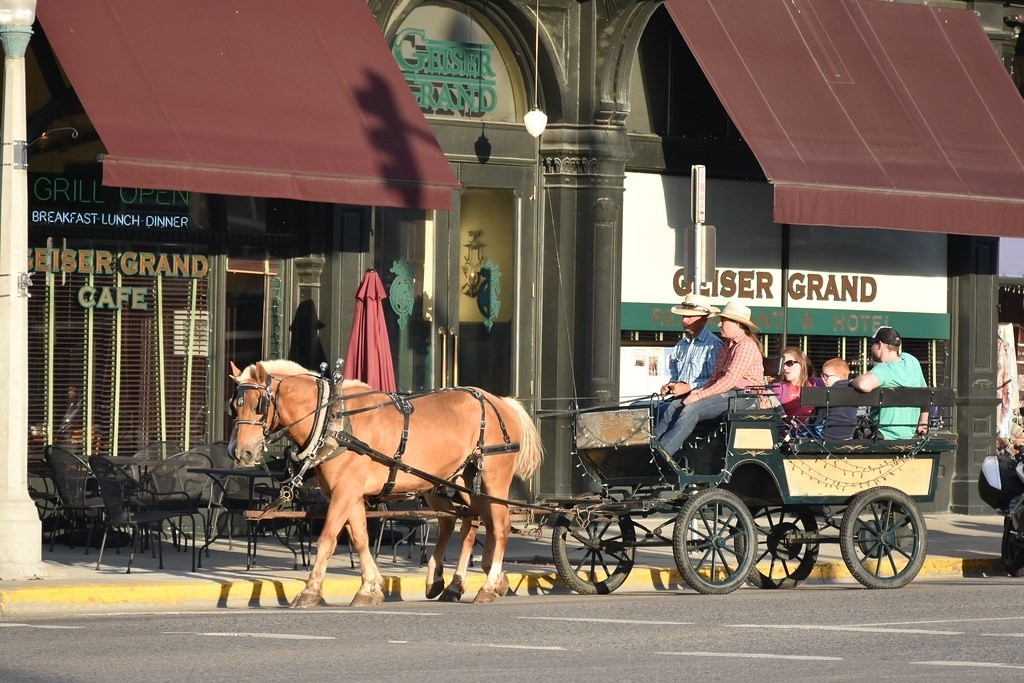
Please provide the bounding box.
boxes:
[849,326,929,440]
[790,357,858,440]
[768,347,818,423]
[628,292,765,458]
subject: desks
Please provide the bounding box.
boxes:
[28,457,202,568]
[186,469,290,572]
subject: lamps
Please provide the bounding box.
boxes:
[523,0,548,138]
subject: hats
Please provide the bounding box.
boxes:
[868,325,901,347]
[716,301,761,332]
[671,294,721,318]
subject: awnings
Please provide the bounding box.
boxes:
[36,0,462,211]
[663,0,1024,237]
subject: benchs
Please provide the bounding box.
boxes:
[662,393,780,422]
[779,388,957,455]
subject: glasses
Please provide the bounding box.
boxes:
[783,359,799,367]
[823,373,835,380]
[681,302,699,308]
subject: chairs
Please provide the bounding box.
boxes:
[27,442,472,574]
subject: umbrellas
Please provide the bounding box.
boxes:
[342,268,398,393]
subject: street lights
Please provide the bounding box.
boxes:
[0,0,42,580]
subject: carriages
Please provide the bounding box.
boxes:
[228,359,959,609]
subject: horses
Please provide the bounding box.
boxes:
[227,358,545,611]
